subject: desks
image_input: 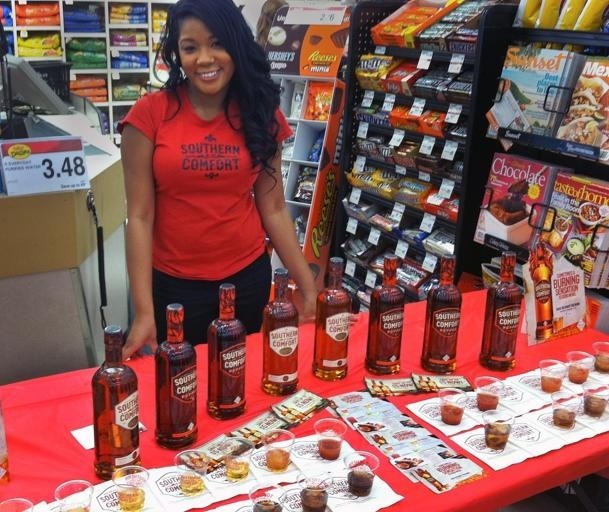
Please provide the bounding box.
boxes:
[0,288,609,512]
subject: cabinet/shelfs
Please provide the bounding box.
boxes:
[470,26,609,299]
[324,0,516,312]
[0,0,186,147]
[270,74,346,313]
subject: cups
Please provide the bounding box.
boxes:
[109,466,152,512]
[176,449,211,496]
[245,482,288,510]
[593,342,609,375]
[311,416,349,461]
[221,437,255,480]
[343,452,382,495]
[549,390,581,430]
[261,430,297,471]
[563,348,593,385]
[435,384,469,426]
[52,476,95,512]
[469,372,503,410]
[537,358,567,394]
[552,317,564,334]
[580,384,609,419]
[293,479,331,510]
[481,410,516,452]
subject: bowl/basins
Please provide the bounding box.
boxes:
[554,202,609,256]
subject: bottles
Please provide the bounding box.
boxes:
[261,267,301,400]
[90,322,145,477]
[208,283,249,424]
[478,251,523,372]
[368,251,402,375]
[420,253,458,375]
[154,300,198,448]
[311,255,351,381]
[532,243,554,340]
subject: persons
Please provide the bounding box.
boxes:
[118,1,359,361]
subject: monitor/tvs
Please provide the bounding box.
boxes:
[0,52,72,119]
